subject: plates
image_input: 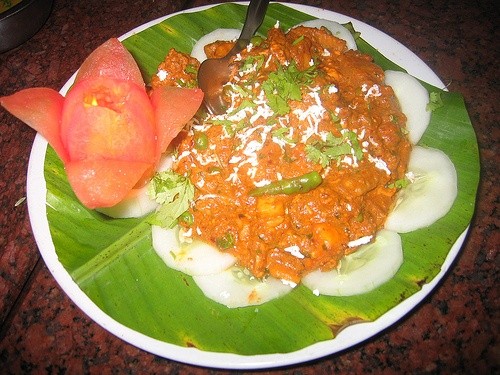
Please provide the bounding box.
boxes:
[27,2,480,371]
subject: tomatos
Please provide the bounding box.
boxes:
[0,37,207,212]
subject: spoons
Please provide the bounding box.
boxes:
[197,0,269,114]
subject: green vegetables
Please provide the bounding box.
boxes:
[143,36,413,230]
